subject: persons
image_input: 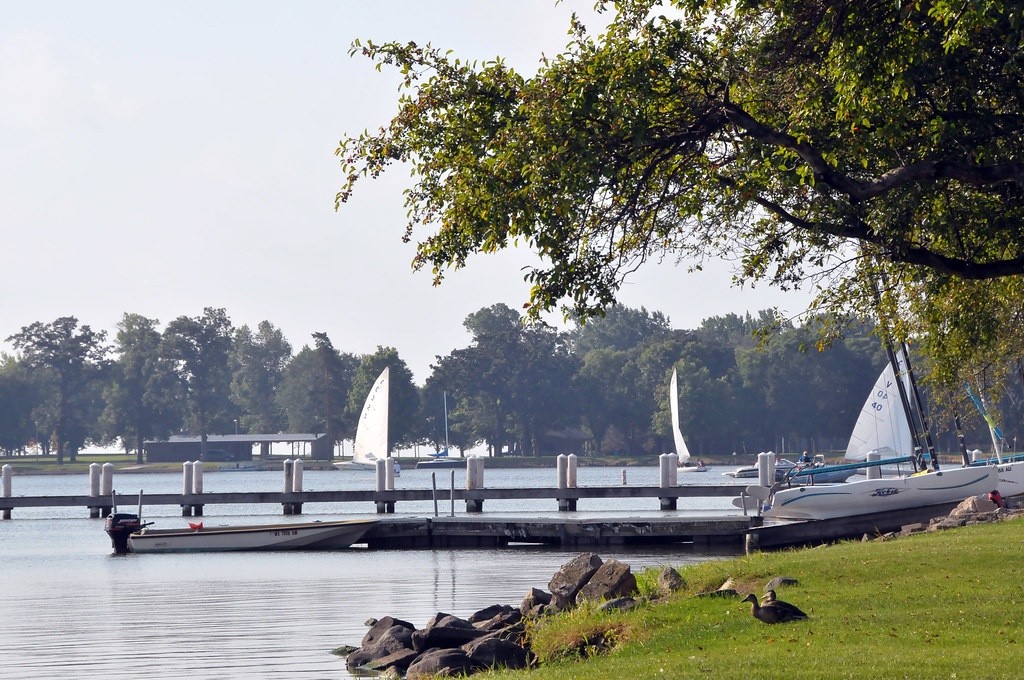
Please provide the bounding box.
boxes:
[697,459,704,467]
[798,451,811,462]
[393,459,401,474]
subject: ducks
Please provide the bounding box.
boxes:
[760,590,809,622]
[740,594,797,624]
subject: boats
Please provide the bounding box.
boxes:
[776,453,856,482]
[104,490,381,550]
[721,459,797,478]
[414,448,466,468]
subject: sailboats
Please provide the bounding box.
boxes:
[962,380,1023,465]
[669,367,711,472]
[845,337,927,476]
[732,237,999,514]
[333,367,396,472]
[944,376,1023,498]
